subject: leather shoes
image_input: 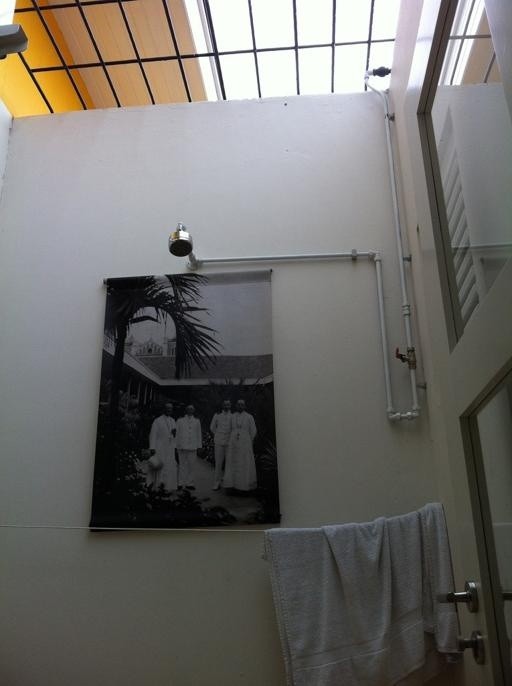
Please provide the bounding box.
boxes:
[185,483,195,491]
[212,481,219,490]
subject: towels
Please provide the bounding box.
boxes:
[263,502,463,686]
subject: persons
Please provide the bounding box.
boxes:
[151,403,179,496]
[211,399,233,492]
[221,398,261,501]
[175,404,204,492]
